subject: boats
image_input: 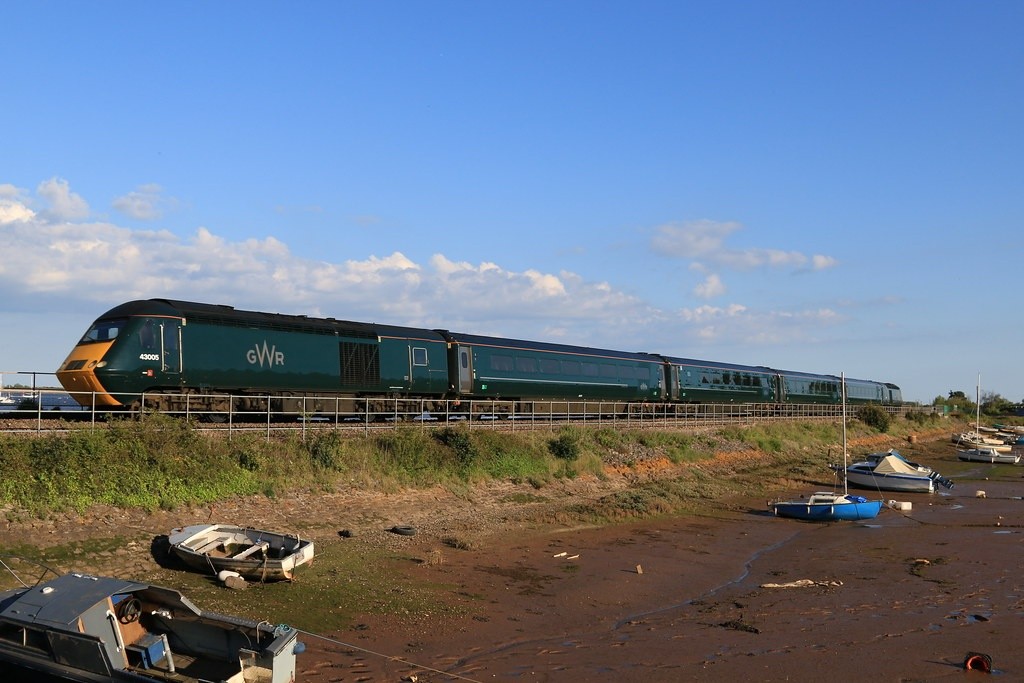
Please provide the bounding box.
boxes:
[952,424,1024,452]
[168,522,316,581]
[0,556,307,683]
[0,390,39,406]
[827,447,955,494]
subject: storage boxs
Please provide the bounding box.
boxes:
[125,633,166,671]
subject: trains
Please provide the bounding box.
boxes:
[54,297,904,421]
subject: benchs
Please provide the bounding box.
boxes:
[196,537,231,554]
[233,541,268,560]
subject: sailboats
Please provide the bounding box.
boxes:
[956,369,1022,464]
[769,372,884,520]
